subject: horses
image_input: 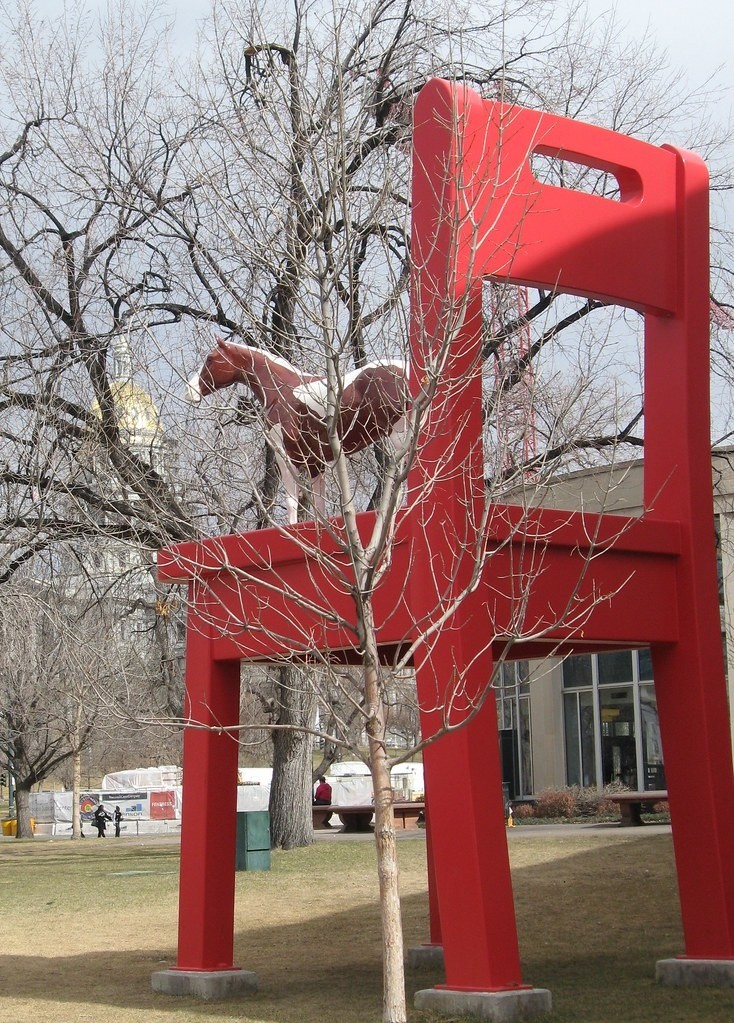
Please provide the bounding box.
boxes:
[184,332,410,526]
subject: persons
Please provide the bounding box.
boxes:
[73,811,85,838]
[114,806,124,837]
[313,776,332,805]
[95,805,112,838]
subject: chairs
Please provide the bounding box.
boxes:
[151,76,734,1023]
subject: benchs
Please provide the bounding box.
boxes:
[310,802,426,831]
[604,791,668,827]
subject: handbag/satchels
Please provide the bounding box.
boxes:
[91,818,98,826]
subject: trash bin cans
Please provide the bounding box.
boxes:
[502,782,510,826]
[1,819,11,836]
[10,818,18,836]
